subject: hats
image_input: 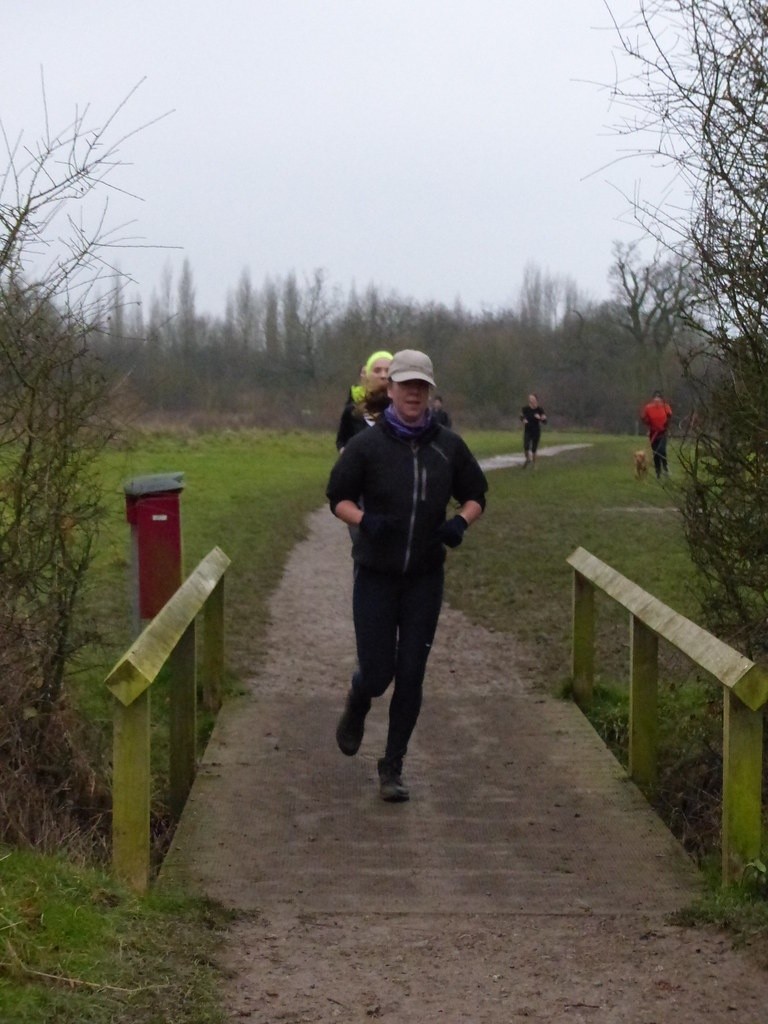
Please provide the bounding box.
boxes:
[388,352,437,390]
[359,351,393,379]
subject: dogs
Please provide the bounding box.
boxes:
[633,451,648,480]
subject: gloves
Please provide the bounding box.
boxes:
[435,514,467,547]
[359,513,394,546]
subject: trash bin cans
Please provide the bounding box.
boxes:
[122,470,186,640]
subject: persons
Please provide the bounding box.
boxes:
[641,391,673,479]
[520,394,547,467]
[324,350,490,800]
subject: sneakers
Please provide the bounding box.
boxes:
[337,687,371,756]
[378,756,410,801]
